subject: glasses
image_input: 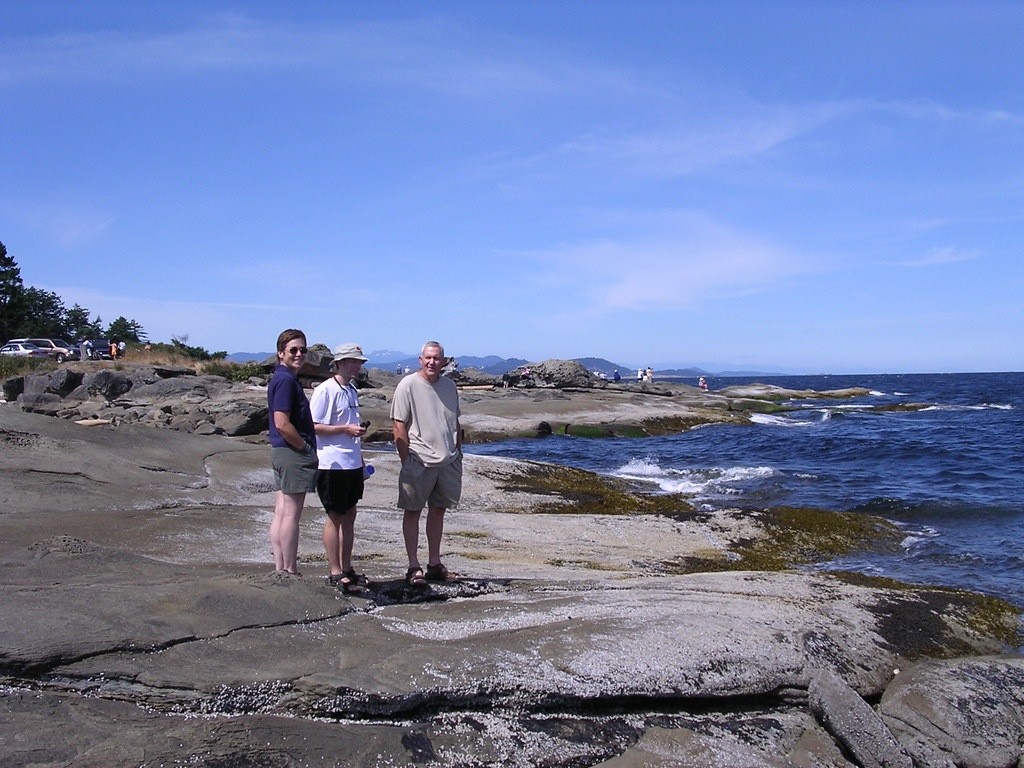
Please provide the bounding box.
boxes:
[285,347,308,354]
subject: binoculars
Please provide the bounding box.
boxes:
[359,420,372,429]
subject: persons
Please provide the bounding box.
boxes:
[593,370,599,377]
[613,369,621,383]
[266,329,319,576]
[107,341,118,360]
[390,341,463,585]
[599,371,606,379]
[698,377,709,391]
[117,339,126,360]
[81,338,94,357]
[647,367,654,382]
[144,340,151,351]
[525,366,530,374]
[396,363,402,374]
[502,372,510,388]
[404,365,410,373]
[643,374,648,382]
[308,343,373,590]
[637,368,644,383]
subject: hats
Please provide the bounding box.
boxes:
[329,344,369,365]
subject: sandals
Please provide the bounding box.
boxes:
[343,566,374,595]
[425,564,461,584]
[406,567,429,590]
[327,570,361,595]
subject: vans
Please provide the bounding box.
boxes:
[5,338,80,363]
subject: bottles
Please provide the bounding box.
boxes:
[362,464,375,480]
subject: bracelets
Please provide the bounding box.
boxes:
[303,443,311,451]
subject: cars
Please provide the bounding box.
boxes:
[0,343,49,358]
[75,335,113,360]
